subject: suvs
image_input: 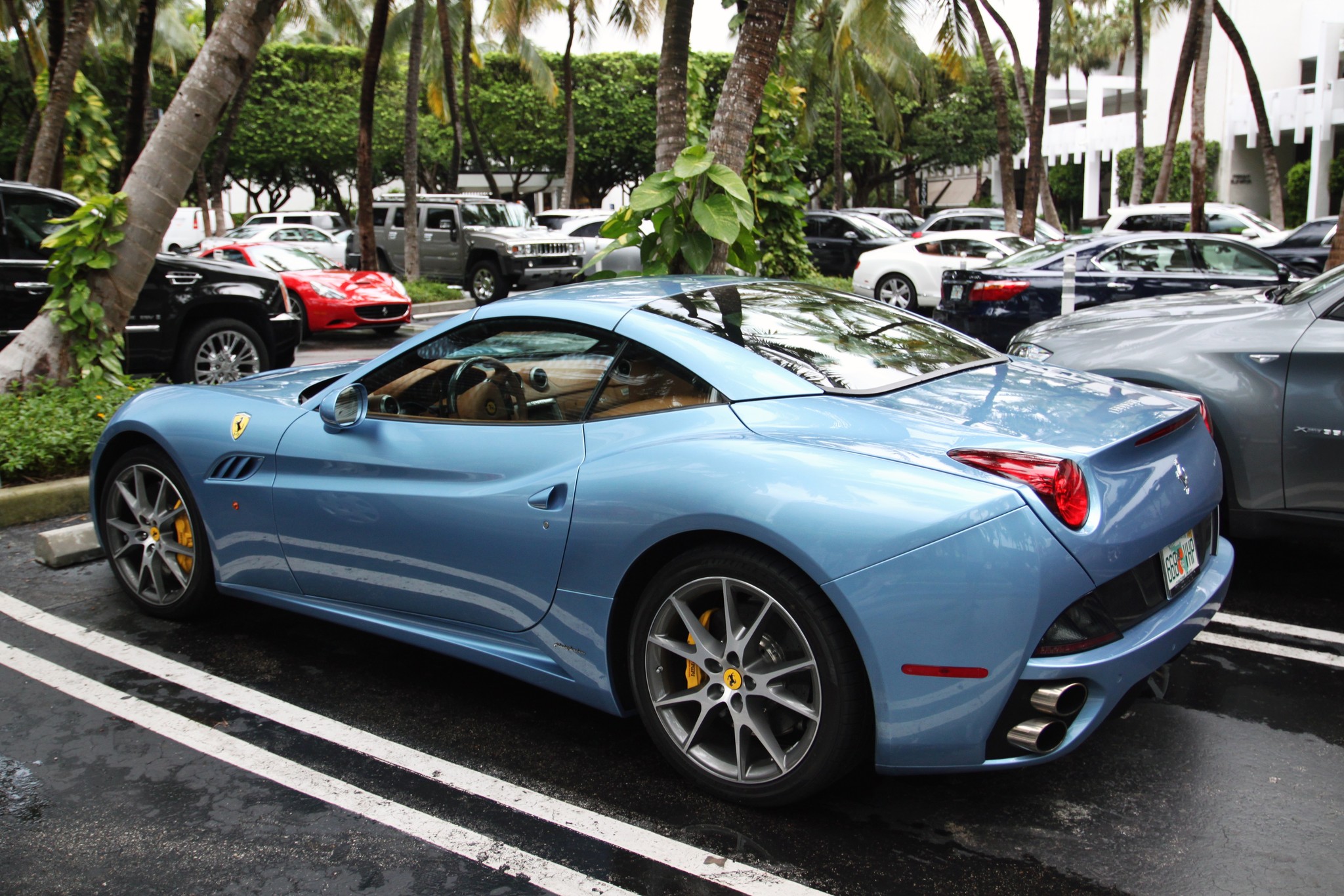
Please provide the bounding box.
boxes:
[841,207,921,241]
[351,192,586,304]
[1094,202,1283,245]
[0,179,304,387]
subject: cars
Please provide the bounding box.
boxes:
[754,210,926,278]
[190,221,349,270]
[191,242,412,339]
[1005,264,1344,530]
[911,207,1065,244]
[557,216,761,276]
[930,230,1330,357]
[533,205,624,232]
[1250,214,1338,275]
[847,229,1039,314]
[158,204,235,256]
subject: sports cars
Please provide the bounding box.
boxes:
[81,270,1233,804]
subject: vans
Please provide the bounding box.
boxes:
[239,212,345,242]
